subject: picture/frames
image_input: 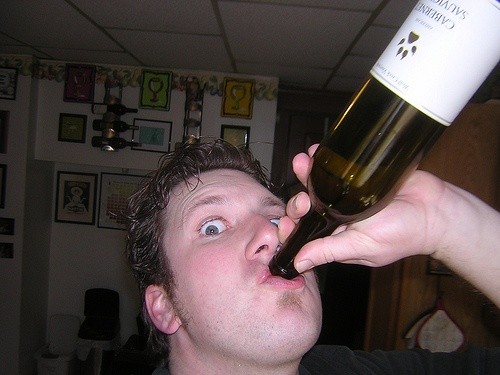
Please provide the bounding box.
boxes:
[63,62,96,104]
[140,70,173,109]
[0,66,18,100]
[221,124,250,150]
[97,172,152,231]
[221,76,255,120]
[132,117,173,153]
[58,112,86,143]
[55,171,98,225]
[0,110,15,258]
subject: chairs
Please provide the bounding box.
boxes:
[77,287,121,375]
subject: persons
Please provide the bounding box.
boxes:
[127,137,500,375]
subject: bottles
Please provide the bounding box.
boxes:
[91,136,142,147]
[91,102,138,115]
[92,119,140,131]
[269,0,500,281]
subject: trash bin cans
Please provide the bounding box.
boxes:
[34,313,81,375]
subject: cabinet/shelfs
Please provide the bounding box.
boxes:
[184,81,204,149]
[100,78,121,152]
